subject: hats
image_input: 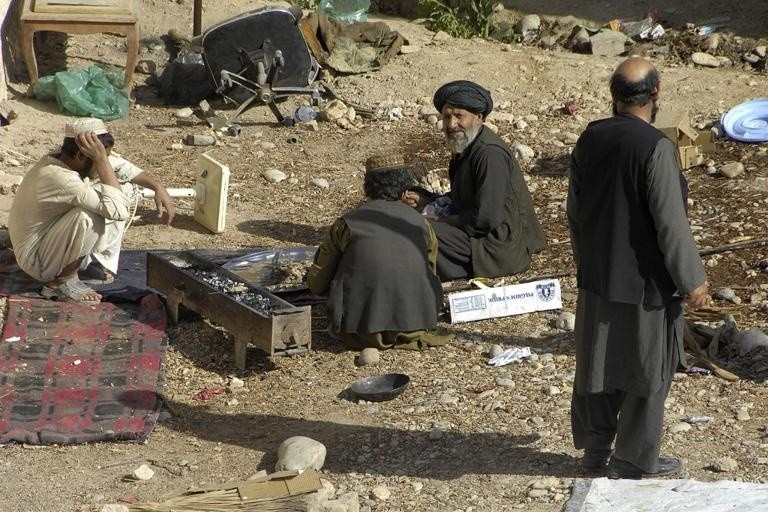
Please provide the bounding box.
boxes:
[366,153,405,173]
[64,117,109,138]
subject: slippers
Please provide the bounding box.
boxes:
[40,276,101,306]
[78,263,114,286]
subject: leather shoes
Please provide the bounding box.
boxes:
[607,456,682,479]
[581,448,614,472]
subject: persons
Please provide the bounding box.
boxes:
[403,82,547,280]
[7,117,175,304]
[305,152,441,347]
[567,58,714,477]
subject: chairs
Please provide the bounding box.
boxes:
[200,4,320,136]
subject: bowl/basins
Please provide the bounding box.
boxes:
[350,373,410,402]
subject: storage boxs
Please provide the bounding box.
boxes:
[650,109,703,172]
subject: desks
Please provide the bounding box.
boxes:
[19,0,139,93]
